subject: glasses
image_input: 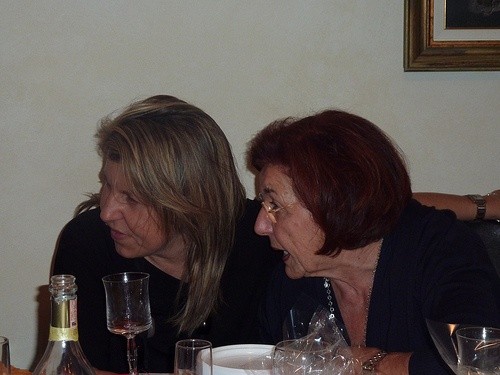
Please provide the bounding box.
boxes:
[255,195,302,226]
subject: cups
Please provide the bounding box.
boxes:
[455,327,500,375]
[273,340,331,375]
[174,339,212,375]
[0,335,11,375]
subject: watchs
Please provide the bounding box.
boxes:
[463,195,487,221]
[361,351,389,375]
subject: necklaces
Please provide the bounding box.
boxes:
[324,237,386,348]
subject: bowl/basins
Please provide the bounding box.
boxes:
[196,344,284,375]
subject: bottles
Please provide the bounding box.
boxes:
[32,275,97,375]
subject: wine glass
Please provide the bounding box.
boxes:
[103,272,152,375]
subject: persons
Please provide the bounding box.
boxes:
[48,94,307,375]
[244,110,500,375]
